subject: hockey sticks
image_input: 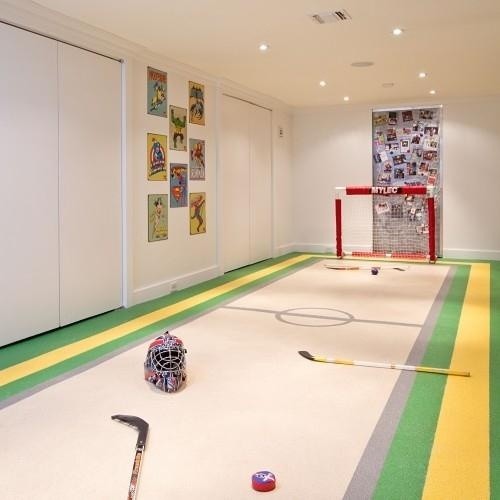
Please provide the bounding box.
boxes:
[111,414,148,500]
[298,351,470,376]
[326,266,405,271]
